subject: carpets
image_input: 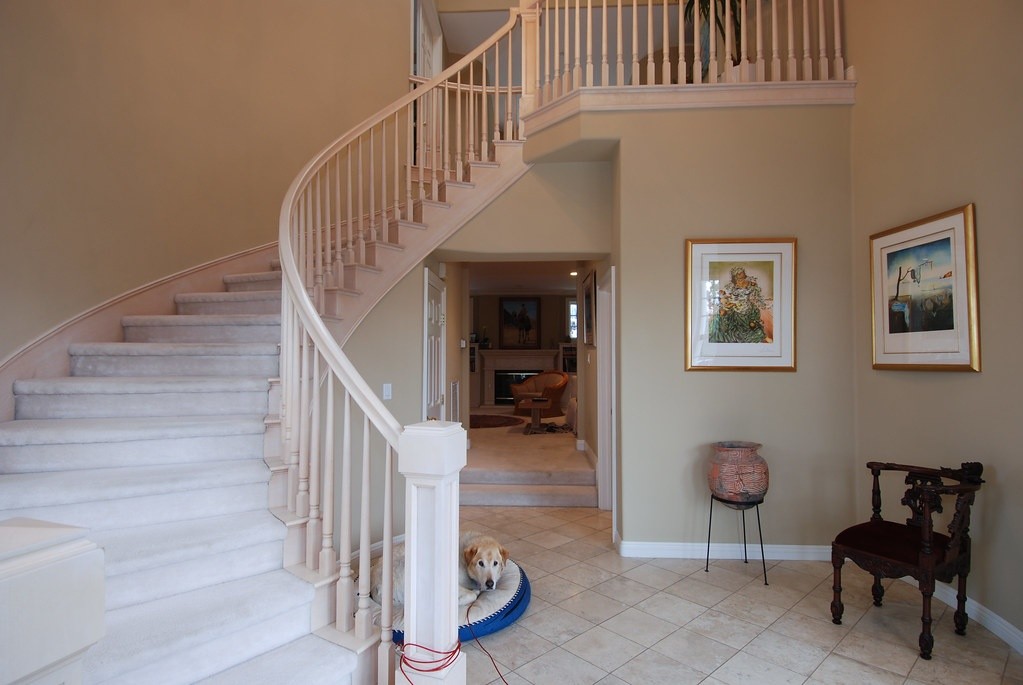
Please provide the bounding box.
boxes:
[470,414,524,428]
[524,421,571,434]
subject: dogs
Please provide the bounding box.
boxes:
[370,530,510,605]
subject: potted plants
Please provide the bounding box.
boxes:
[684,0,757,82]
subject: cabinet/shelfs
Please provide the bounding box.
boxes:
[469,343,483,407]
[558,343,577,374]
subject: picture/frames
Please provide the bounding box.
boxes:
[871,202,981,373]
[685,236,797,371]
[583,269,597,347]
[498,296,541,349]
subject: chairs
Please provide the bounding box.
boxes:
[831,460,985,659]
[511,369,569,416]
